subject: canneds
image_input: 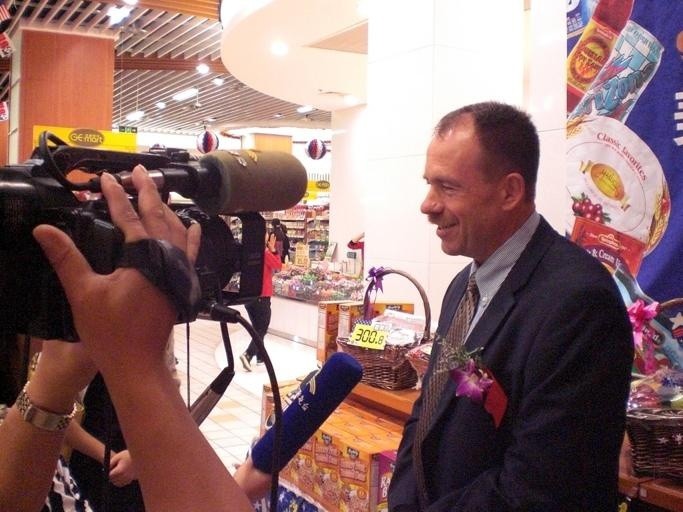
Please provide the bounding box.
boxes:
[566,22,664,123]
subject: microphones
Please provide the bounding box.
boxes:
[250,350,364,476]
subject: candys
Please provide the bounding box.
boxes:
[580,160,632,211]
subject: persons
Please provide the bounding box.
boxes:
[386,101,634,512]
[240,219,290,371]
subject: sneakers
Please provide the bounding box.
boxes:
[240,352,264,372]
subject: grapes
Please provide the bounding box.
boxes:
[572,198,604,223]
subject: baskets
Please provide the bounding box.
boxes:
[337,269,436,390]
[626,297,682,477]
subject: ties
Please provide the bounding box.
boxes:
[412,271,480,507]
